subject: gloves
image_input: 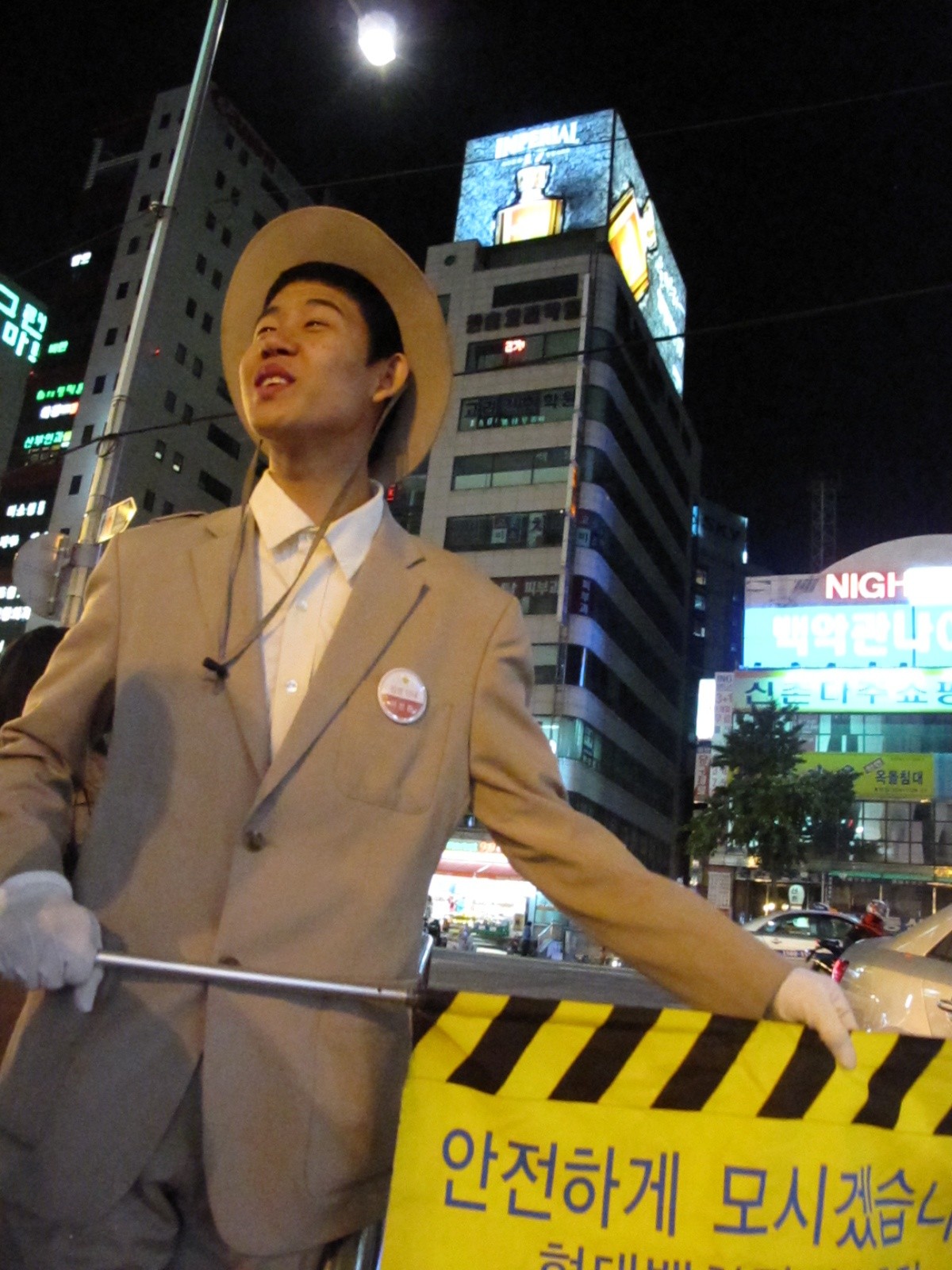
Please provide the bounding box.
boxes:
[765,966,858,1069]
[0,870,104,1011]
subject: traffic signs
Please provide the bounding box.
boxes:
[93,494,135,546]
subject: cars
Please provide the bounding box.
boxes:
[831,904,952,1046]
[741,906,894,970]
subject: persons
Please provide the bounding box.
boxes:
[425,895,441,946]
[844,899,886,948]
[520,921,532,955]
[0,207,857,1270]
[0,626,110,1059]
[599,947,607,965]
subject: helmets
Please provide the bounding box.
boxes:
[866,899,889,923]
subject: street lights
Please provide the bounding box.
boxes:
[59,0,399,634]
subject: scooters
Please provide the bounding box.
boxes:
[809,937,843,976]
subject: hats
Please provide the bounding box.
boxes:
[221,206,454,490]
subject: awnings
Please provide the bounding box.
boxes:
[476,865,527,881]
[434,860,485,878]
